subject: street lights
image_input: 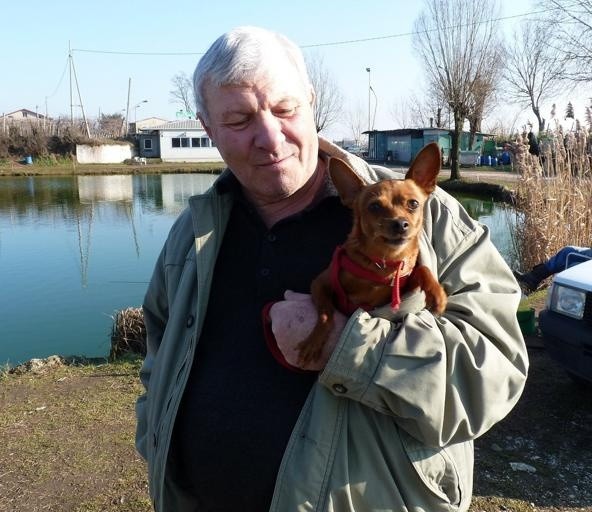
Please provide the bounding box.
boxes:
[366,68,371,131]
[134,99,149,122]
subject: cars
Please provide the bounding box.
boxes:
[538,259,592,389]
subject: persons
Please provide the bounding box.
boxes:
[133,24,532,512]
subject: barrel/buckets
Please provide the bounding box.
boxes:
[24,155,32,164]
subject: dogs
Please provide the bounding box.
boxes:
[291,141,449,370]
